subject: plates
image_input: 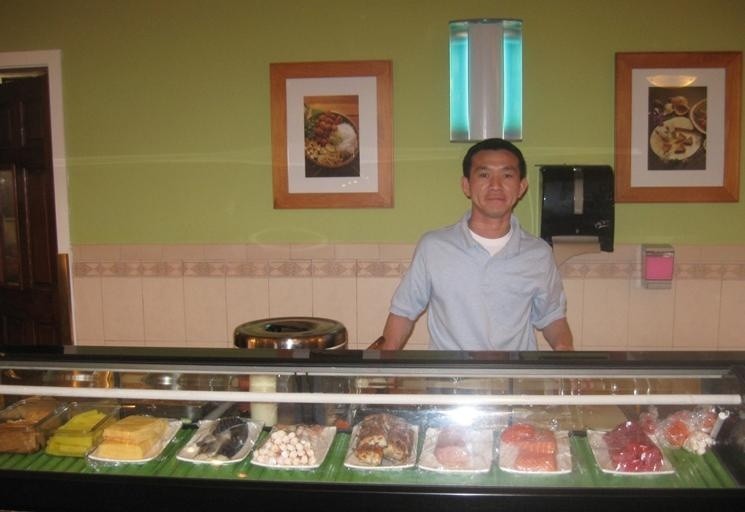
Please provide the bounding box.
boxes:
[342,423,419,471]
[498,429,573,475]
[250,426,337,472]
[88,418,183,465]
[174,418,265,465]
[649,98,706,161]
[416,427,494,475]
[586,429,676,475]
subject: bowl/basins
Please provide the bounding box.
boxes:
[304,111,359,169]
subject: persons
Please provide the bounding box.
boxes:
[378,137,575,351]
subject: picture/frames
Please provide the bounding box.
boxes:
[613,50,743,203]
[269,60,393,208]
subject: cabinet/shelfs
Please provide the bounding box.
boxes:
[0,349,745,511]
